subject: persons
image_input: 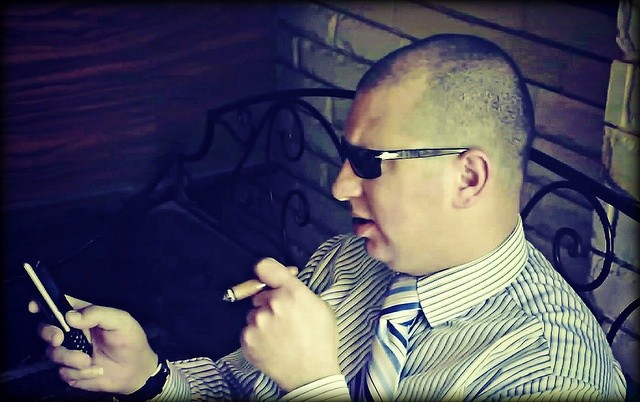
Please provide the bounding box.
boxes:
[27,33,628,402]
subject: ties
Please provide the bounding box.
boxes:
[366,274,423,401]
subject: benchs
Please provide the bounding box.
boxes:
[1,89,637,402]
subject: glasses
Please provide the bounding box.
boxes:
[341,137,469,179]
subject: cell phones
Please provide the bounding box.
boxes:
[23,253,94,360]
